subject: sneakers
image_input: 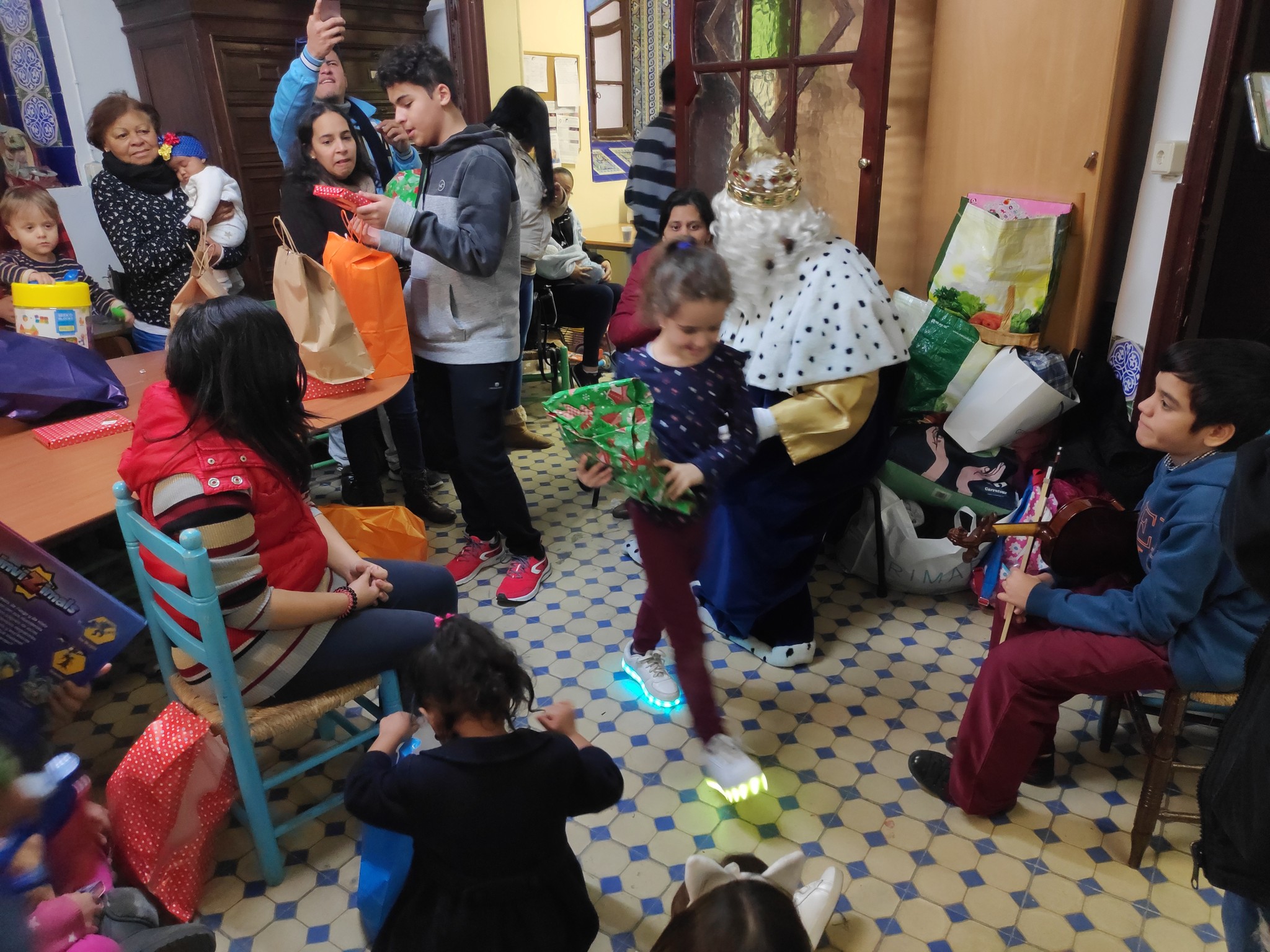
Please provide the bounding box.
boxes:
[495,547,552,602]
[444,530,507,585]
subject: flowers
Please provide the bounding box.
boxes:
[158,132,180,160]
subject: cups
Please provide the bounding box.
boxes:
[621,226,633,242]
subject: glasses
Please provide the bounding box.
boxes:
[294,34,341,60]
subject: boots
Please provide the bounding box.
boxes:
[503,405,554,448]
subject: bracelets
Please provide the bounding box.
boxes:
[333,586,358,618]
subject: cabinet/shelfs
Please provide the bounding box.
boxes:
[113,0,429,302]
[774,0,1147,355]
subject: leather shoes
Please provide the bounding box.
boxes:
[907,749,1017,819]
[945,735,1055,789]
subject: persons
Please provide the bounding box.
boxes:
[609,185,716,522]
[908,331,1270,952]
[345,614,624,952]
[574,236,770,804]
[623,145,911,669]
[624,62,677,267]
[0,760,217,952]
[649,851,844,952]
[116,295,459,725]
[0,0,623,603]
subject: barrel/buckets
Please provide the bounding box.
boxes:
[11,280,95,352]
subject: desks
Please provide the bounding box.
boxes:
[92,314,123,339]
[582,223,637,285]
[0,350,410,543]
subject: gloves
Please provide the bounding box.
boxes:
[719,407,780,445]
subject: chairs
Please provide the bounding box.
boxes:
[113,481,403,886]
[1098,686,1239,869]
[540,284,612,357]
[520,330,569,405]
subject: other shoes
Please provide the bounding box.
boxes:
[700,734,768,805]
[361,485,385,508]
[620,642,680,708]
[405,492,458,524]
[792,868,844,951]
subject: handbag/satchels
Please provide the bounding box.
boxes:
[356,689,442,949]
[271,215,376,385]
[165,219,229,356]
[322,208,414,379]
[833,184,1125,614]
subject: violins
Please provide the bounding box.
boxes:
[947,495,1141,582]
